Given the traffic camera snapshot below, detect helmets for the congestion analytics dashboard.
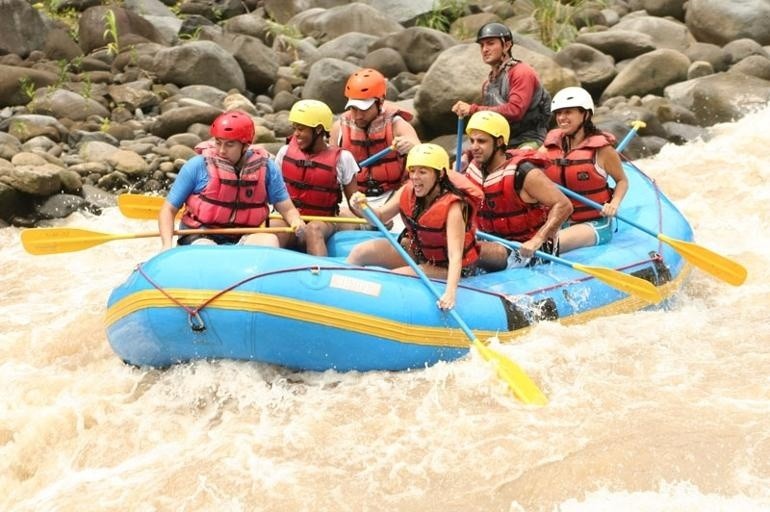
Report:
[465,110,510,167]
[208,111,255,168]
[288,99,334,154]
[549,86,596,139]
[344,67,387,129]
[406,143,450,199]
[475,21,514,65]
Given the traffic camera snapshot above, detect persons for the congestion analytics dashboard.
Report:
[329,68,423,197]
[342,140,483,312]
[508,84,632,252]
[459,109,577,273]
[449,20,556,153]
[155,108,306,252]
[261,98,395,260]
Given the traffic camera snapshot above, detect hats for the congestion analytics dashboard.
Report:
[344,96,378,111]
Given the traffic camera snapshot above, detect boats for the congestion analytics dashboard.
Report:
[105,154,699,375]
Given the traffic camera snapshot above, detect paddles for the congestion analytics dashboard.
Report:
[117,193,366,223]
[547,183,748,287]
[355,194,550,409]
[21,228,300,256]
[475,229,663,304]
